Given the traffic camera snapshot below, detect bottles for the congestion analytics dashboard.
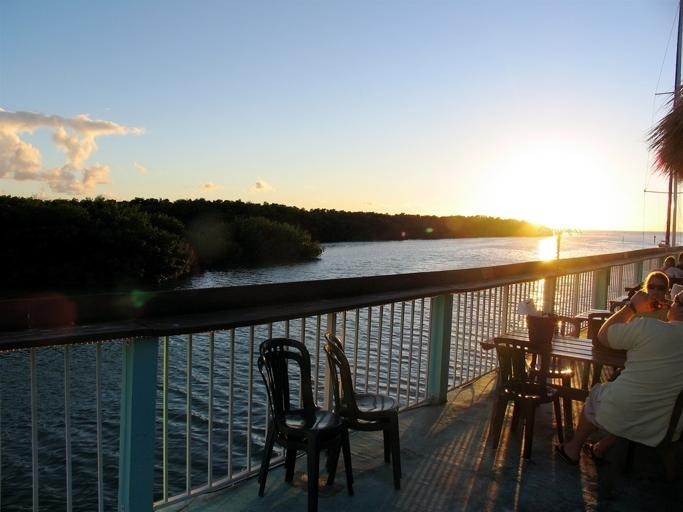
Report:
[628,288,670,314]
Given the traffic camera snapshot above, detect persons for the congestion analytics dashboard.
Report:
[661,256,682,279]
[676,253,682,269]
[623,270,672,324]
[550,285,682,468]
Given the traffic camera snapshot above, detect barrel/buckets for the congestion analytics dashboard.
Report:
[526,312,557,342]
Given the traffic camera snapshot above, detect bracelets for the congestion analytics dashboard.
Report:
[624,298,637,316]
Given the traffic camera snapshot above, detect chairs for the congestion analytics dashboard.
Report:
[256,333,403,511]
[490,275,682,480]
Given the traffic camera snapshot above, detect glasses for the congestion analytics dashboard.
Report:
[648,285,666,290]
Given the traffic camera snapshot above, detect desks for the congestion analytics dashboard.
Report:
[481,324,627,426]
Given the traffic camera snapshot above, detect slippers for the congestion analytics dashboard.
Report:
[583,441,602,464]
[556,442,577,463]
